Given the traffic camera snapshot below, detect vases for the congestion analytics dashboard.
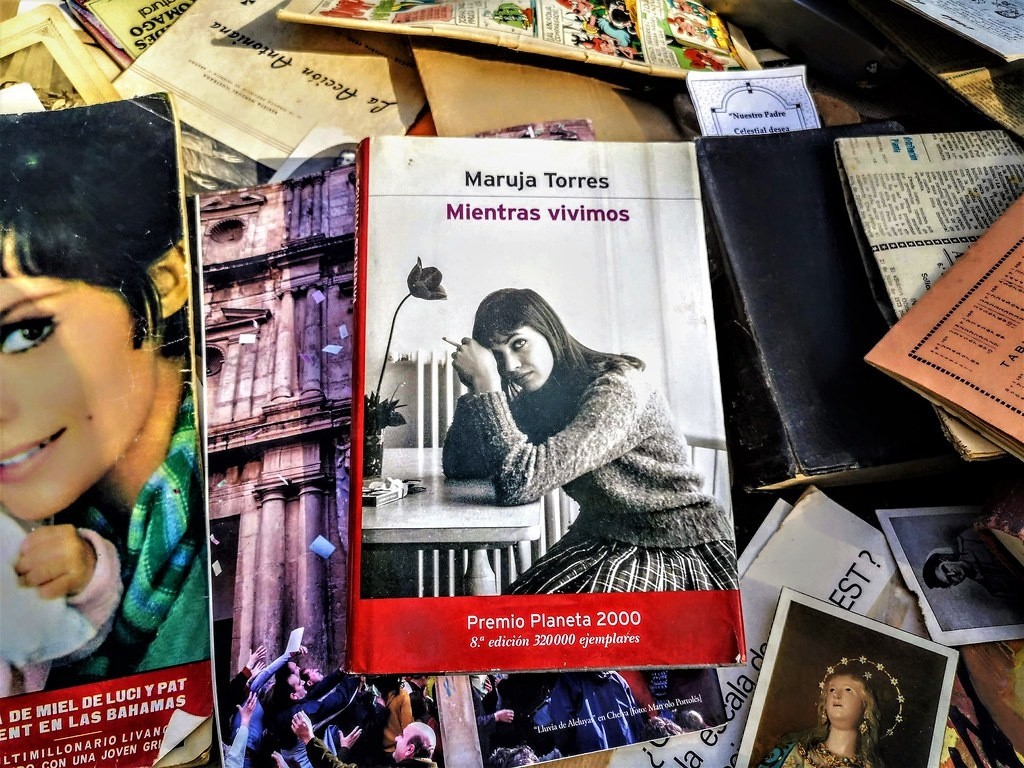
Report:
[362,431,384,487]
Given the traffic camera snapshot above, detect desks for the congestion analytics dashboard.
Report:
[363,448,543,597]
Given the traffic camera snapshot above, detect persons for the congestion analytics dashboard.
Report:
[221,645,442,768]
[0,115,209,698]
[471,673,708,768]
[335,149,357,167]
[444,292,739,595]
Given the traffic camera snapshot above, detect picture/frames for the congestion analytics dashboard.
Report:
[0,2,122,111]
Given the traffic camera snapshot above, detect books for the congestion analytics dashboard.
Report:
[346,135,747,674]
[691,128,1024,494]
[0,92,222,768]
[278,0,761,80]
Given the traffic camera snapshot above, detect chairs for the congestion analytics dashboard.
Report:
[686,434,734,529]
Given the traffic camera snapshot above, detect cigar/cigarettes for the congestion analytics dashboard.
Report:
[442,336,460,348]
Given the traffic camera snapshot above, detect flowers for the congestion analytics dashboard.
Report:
[363,255,448,437]
